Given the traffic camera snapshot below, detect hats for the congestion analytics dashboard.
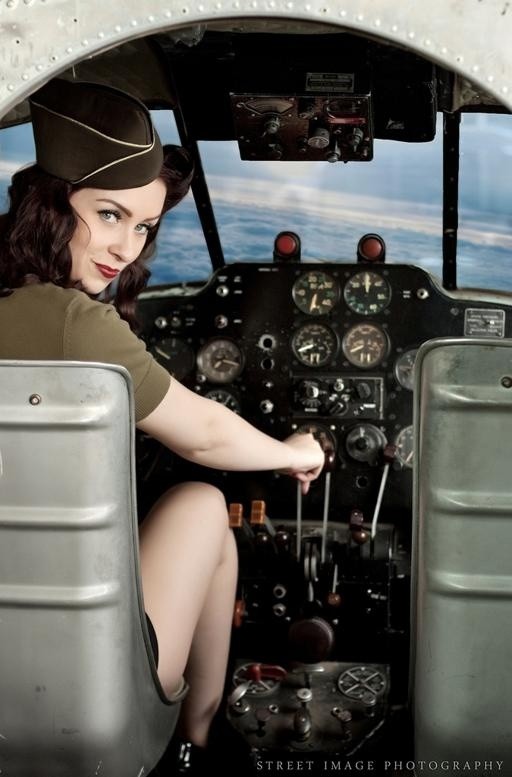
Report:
[27,75,165,194]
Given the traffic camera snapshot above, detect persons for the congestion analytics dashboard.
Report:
[1,76,325,776]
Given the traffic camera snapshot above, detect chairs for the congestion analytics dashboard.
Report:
[404,335,511,777]
[0,358,188,777]
